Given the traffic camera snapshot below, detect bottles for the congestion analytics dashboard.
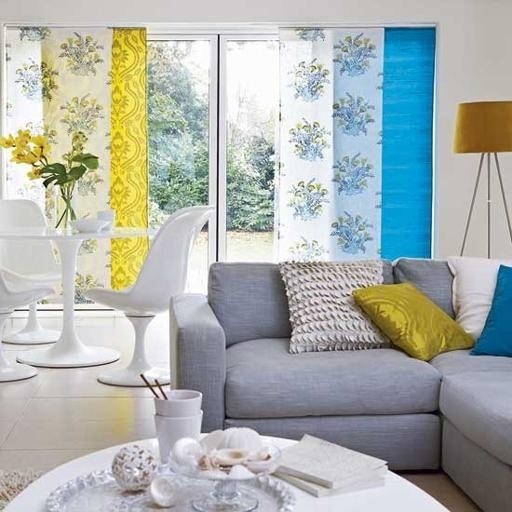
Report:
[112,443,157,494]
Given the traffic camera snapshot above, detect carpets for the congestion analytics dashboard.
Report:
[0,469,44,512]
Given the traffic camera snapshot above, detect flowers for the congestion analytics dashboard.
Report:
[1,125,103,228]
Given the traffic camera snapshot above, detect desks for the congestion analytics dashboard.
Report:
[0,227,159,370]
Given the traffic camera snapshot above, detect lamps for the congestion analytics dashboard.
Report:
[453,100,512,259]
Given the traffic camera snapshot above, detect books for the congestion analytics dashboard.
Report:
[269,432,389,497]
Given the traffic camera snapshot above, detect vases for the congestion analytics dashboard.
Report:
[52,180,79,234]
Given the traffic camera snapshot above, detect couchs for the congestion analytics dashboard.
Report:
[167,256,512,512]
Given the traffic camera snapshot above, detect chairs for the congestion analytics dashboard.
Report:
[0,198,63,346]
[82,204,217,388]
[0,265,57,384]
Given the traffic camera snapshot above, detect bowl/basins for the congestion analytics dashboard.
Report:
[74,218,107,232]
[148,478,181,506]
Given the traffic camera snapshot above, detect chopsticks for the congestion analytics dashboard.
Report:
[140,370,168,398]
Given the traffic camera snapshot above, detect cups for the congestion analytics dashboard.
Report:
[98,209,120,228]
[152,389,203,460]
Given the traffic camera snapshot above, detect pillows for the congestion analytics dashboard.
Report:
[276,259,397,354]
[352,282,475,361]
[446,257,512,344]
[469,264,512,358]
